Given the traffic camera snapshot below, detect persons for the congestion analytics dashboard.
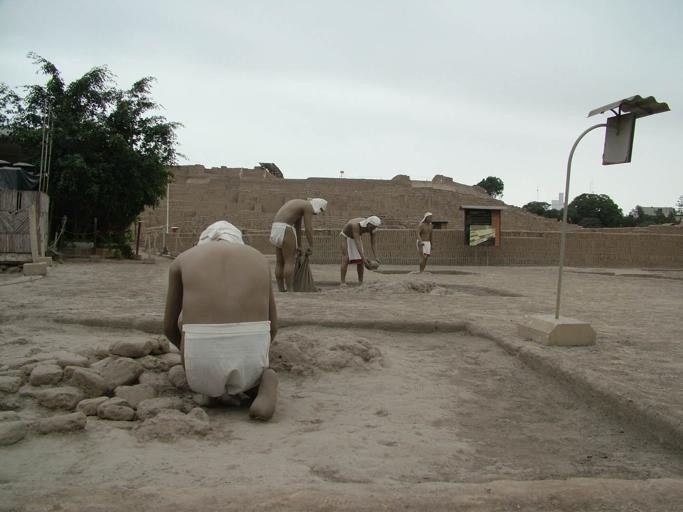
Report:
[337,216,381,288]
[268,197,327,292]
[162,219,278,422]
[415,212,433,272]
